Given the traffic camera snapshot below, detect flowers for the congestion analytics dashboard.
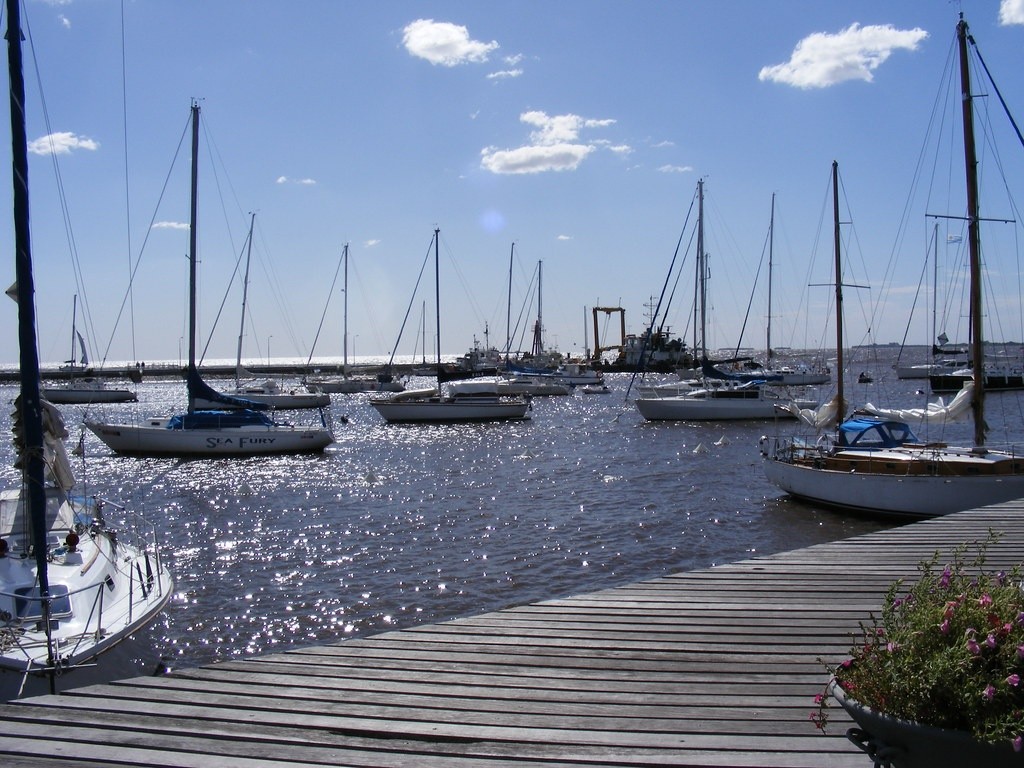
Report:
[809,525,1024,753]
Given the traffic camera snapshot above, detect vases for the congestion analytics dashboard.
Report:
[829,659,1024,768]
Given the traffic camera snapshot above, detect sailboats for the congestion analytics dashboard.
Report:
[368,177,834,425]
[893,213,1023,393]
[300,243,406,393]
[196,212,333,409]
[80,95,336,456]
[1,0,174,707]
[41,295,139,403]
[756,11,1023,519]
